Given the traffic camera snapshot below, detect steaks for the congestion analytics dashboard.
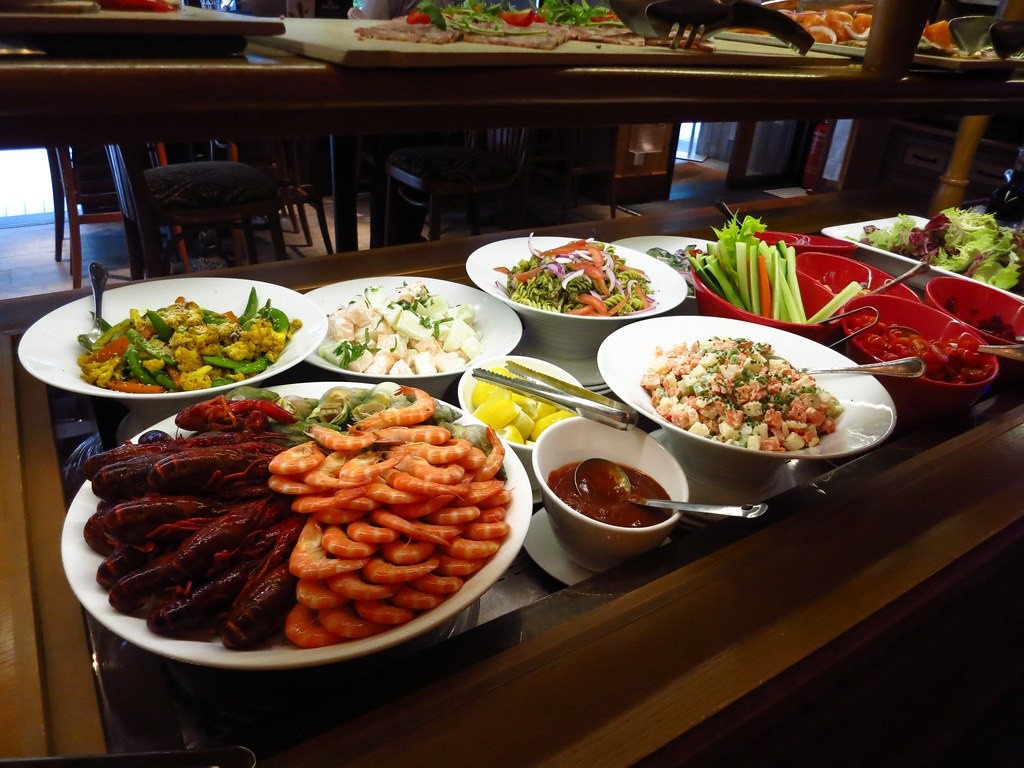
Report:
[349,10,716,51]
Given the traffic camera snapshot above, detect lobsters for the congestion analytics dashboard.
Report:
[82,395,299,652]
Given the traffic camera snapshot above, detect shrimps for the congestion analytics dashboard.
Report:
[270,385,508,647]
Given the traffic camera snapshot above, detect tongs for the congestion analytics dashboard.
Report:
[472,361,642,434]
[809,302,880,352]
[948,10,1024,61]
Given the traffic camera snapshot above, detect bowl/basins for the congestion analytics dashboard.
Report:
[455,356,590,493]
[594,313,900,519]
[531,412,691,575]
[691,211,1024,433]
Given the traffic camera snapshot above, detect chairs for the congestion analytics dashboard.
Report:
[49,144,123,288]
[101,140,294,271]
[379,126,537,240]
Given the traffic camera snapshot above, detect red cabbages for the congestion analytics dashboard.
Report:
[862,180,1024,302]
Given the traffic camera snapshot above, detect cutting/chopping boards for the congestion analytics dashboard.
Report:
[245,13,853,72]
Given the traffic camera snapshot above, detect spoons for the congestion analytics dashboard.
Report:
[887,323,1024,363]
[755,356,926,383]
[78,262,111,356]
[573,459,769,519]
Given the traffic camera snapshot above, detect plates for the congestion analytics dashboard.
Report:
[466,234,689,362]
[290,276,523,379]
[608,236,727,318]
[61,379,534,670]
[16,277,328,420]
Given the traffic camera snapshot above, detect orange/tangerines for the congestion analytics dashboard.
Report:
[727,10,954,48]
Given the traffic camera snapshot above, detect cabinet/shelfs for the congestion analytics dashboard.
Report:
[3,0,1024,768]
